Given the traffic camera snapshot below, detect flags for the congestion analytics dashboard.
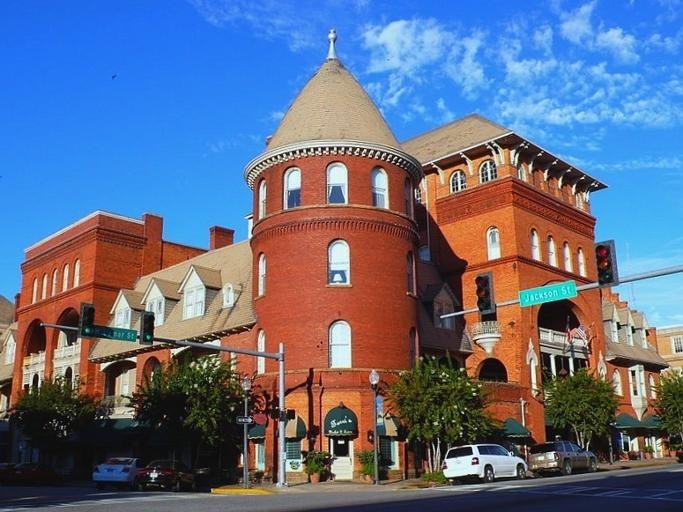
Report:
[566,320,586,344]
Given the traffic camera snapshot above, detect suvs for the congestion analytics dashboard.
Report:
[441,444,529,483]
[527,441,599,477]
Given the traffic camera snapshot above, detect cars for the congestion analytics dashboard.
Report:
[0,462,57,486]
[92,457,200,492]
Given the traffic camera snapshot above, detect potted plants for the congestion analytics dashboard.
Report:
[356,448,385,484]
[643,446,655,460]
[669,447,680,458]
[300,449,332,484]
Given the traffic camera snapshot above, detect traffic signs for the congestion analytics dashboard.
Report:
[102,326,137,342]
[518,280,577,308]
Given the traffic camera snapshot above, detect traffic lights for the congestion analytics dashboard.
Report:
[474,271,495,315]
[594,239,619,287]
[77,303,96,338]
[139,311,154,345]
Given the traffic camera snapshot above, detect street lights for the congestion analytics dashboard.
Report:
[368,369,380,485]
[241,378,252,488]
